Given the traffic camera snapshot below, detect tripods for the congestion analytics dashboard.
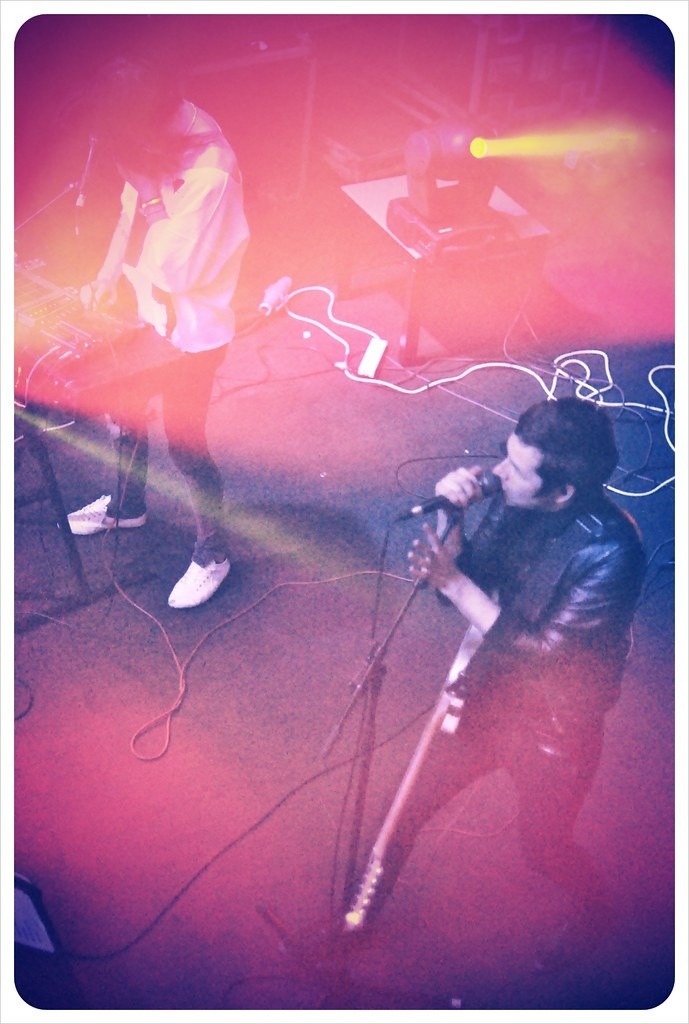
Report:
[250,504,467,1009]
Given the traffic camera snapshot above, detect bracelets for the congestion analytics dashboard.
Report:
[145,206,165,220]
[141,196,163,209]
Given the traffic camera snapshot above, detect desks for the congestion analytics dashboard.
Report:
[338,168,554,369]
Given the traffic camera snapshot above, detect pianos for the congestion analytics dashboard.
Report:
[14,261,186,423]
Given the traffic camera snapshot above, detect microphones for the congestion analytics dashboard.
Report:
[73,127,104,210]
[396,469,503,521]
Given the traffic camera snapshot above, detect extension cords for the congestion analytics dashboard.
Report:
[358,334,388,379]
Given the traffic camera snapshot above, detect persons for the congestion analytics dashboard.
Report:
[344,396,649,970]
[50,51,250,608]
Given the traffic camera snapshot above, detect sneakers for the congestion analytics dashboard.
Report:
[167,557,231,608]
[58,494,147,535]
